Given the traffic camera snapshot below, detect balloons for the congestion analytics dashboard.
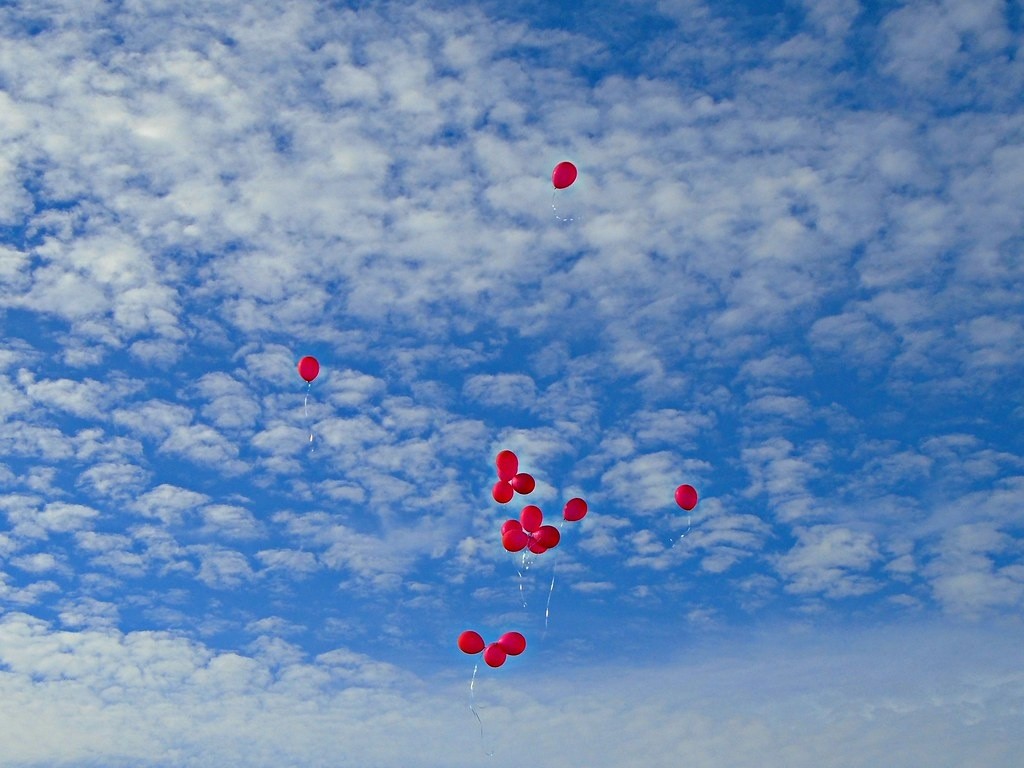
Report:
[492,482,514,503]
[526,533,548,555]
[553,161,578,190]
[496,450,519,479]
[484,643,507,667]
[497,469,518,482]
[520,505,543,533]
[498,631,526,656]
[533,525,560,549]
[502,529,528,552]
[564,498,588,521]
[510,473,535,494]
[675,484,698,511]
[501,520,522,535]
[298,356,320,383]
[458,630,485,654]
